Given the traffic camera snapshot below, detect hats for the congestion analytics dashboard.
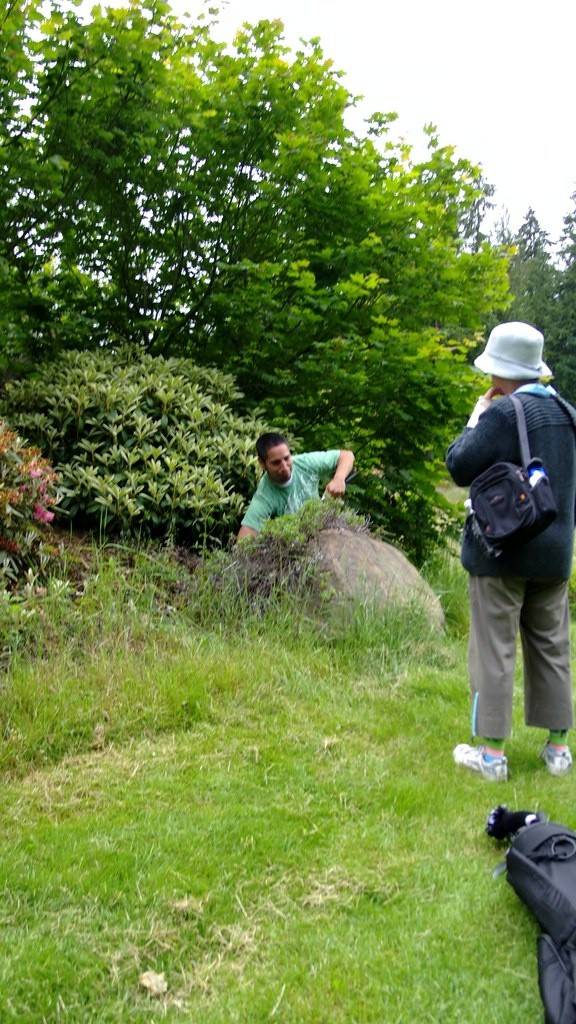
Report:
[474,321,552,380]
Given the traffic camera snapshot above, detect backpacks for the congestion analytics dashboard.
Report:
[506,817,576,1024]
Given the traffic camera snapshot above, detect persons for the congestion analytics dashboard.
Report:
[445,322,576,783]
[235,432,356,544]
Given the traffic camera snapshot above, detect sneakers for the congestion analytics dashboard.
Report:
[539,739,572,777]
[453,744,507,782]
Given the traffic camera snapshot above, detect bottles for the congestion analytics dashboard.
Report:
[529,466,556,514]
[463,498,496,560]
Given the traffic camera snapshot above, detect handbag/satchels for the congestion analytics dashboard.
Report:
[463,391,556,561]
